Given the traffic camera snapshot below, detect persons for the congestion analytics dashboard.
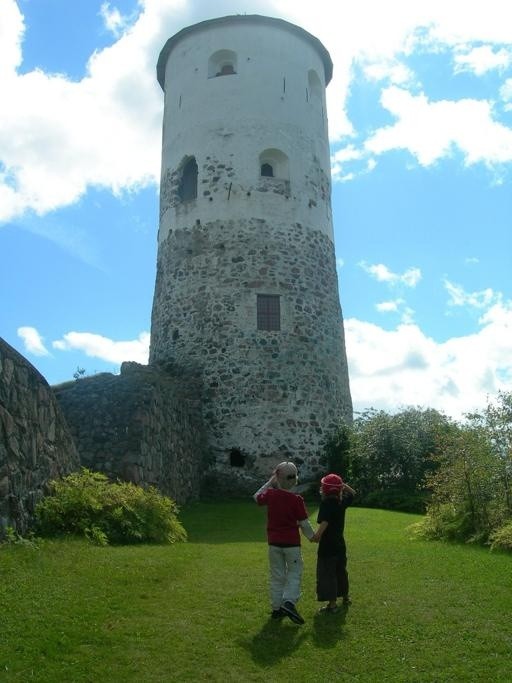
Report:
[315,474,356,613]
[253,462,315,624]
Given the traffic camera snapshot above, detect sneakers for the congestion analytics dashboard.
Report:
[279,601,306,625]
[270,609,287,619]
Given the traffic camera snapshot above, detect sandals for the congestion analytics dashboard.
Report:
[317,605,340,616]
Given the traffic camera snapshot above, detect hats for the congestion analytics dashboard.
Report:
[320,473,344,494]
[274,459,298,490]
